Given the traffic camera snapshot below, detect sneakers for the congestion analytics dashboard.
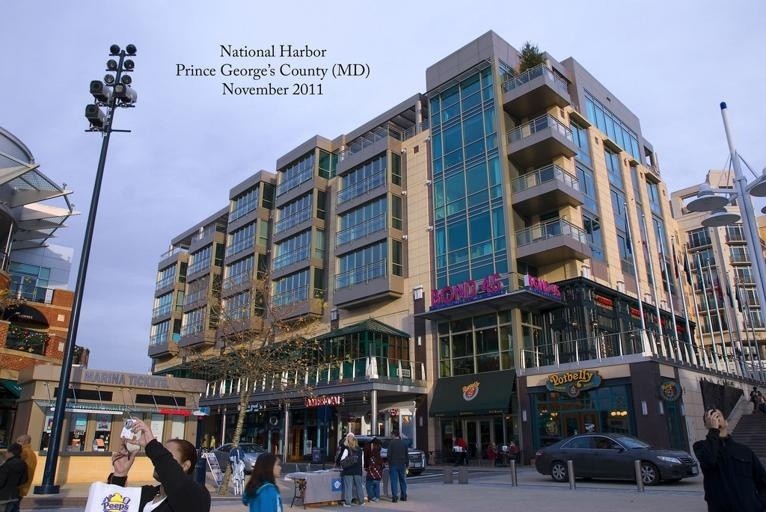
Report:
[343,496,409,508]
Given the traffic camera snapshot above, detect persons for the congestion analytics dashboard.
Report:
[339,437,365,506]
[241,452,283,512]
[0,442,29,512]
[331,433,353,470]
[454,434,469,465]
[208,434,215,452]
[508,439,520,455]
[199,433,207,449]
[691,408,766,512]
[106,416,210,511]
[749,386,766,414]
[487,444,496,469]
[365,438,382,503]
[13,434,37,504]
[386,430,409,503]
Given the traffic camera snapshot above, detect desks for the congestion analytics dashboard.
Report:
[288,470,367,509]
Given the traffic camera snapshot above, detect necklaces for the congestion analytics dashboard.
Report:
[154,491,159,497]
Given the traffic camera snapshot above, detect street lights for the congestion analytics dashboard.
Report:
[34,44,137,495]
[686,101,766,329]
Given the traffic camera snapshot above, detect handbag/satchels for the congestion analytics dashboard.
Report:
[339,454,360,470]
[84,472,143,512]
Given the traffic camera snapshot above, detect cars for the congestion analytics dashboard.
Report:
[205,443,266,473]
[337,435,425,476]
[535,432,699,485]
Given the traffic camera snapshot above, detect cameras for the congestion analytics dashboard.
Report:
[120,419,142,442]
